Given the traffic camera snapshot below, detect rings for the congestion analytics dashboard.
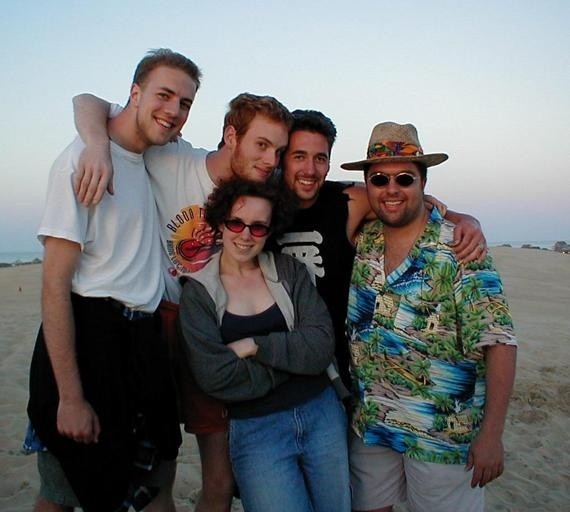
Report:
[478,241,484,253]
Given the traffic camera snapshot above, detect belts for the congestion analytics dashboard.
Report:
[109,300,152,323]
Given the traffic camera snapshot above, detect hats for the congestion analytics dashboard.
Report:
[340,122,449,170]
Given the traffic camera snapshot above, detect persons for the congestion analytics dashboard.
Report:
[70,89,448,510]
[17,46,206,510]
[177,176,354,511]
[260,108,488,407]
[337,120,519,510]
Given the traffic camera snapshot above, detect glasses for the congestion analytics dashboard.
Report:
[222,218,274,238]
[366,171,421,188]
